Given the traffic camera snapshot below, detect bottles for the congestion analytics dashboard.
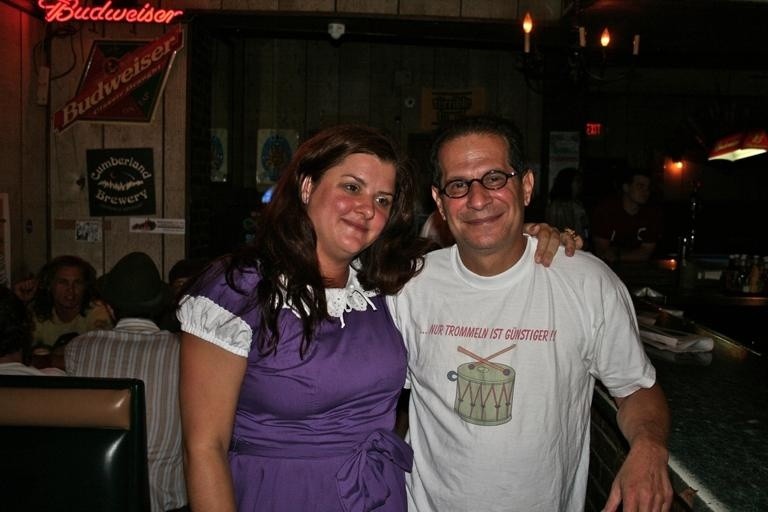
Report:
[726,253,768,298]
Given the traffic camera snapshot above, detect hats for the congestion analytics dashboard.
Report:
[94,253,172,316]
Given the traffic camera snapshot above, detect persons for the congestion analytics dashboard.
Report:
[2,251,215,512]
[416,207,458,252]
[542,164,593,250]
[375,109,683,511]
[167,121,584,511]
[586,164,671,264]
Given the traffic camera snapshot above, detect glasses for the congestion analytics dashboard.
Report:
[436,171,517,199]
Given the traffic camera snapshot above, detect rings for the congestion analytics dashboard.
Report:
[569,229,578,240]
